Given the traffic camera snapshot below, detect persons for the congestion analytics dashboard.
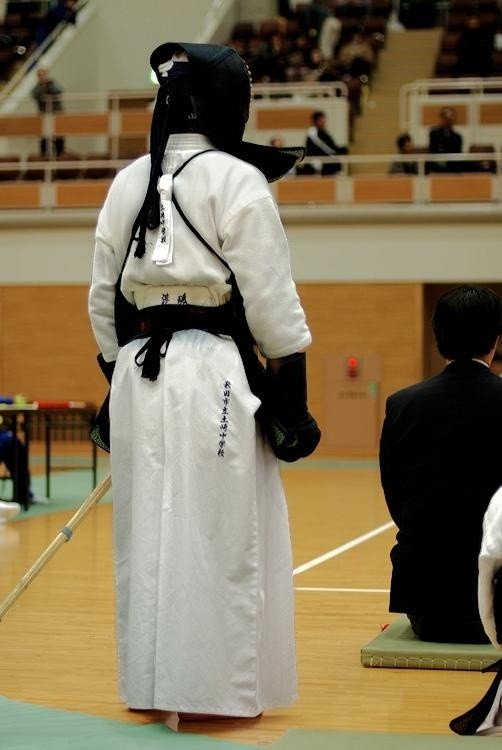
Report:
[375,278,502,648]
[302,106,350,178]
[269,134,301,179]
[0,0,86,83]
[425,106,463,173]
[87,43,323,725]
[224,0,502,142]
[0,397,52,512]
[390,133,415,175]
[28,67,70,158]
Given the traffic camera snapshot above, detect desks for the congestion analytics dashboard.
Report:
[0,406,98,497]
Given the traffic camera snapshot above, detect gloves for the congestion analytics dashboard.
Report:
[255,352,321,463]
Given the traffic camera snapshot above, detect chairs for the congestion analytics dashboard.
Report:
[0,3,502,228]
